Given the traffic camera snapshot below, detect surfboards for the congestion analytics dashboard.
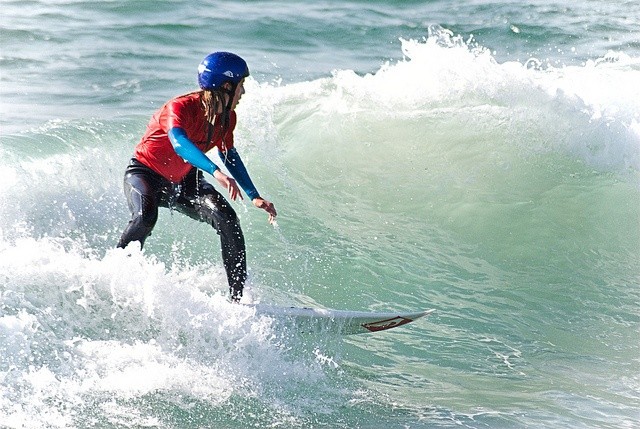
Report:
[242,304,437,335]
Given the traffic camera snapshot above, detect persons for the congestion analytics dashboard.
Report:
[117,52,276,303]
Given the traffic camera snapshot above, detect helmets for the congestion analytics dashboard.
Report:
[197,52,249,89]
